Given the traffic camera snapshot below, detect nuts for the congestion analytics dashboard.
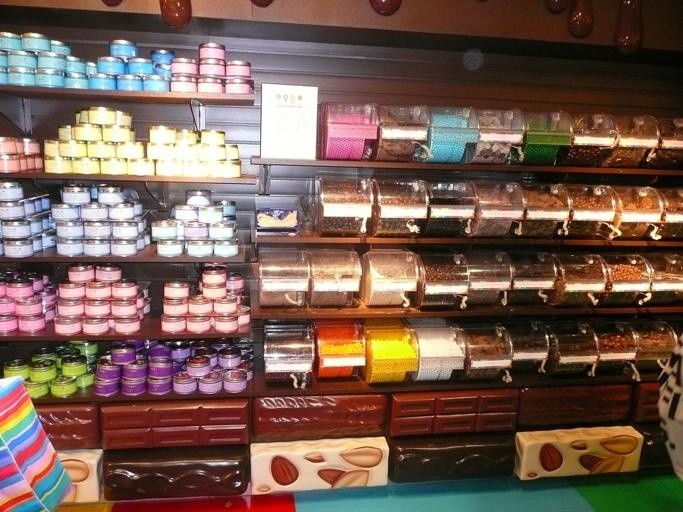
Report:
[60,459,89,482]
[339,446,383,467]
[257,485,270,492]
[539,442,563,472]
[527,472,537,477]
[600,435,638,454]
[63,484,77,502]
[571,442,587,450]
[271,456,299,486]
[318,469,369,489]
[304,452,325,463]
[579,454,624,475]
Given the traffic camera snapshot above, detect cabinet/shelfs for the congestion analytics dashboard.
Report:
[1,80,253,505]
[252,73,683,498]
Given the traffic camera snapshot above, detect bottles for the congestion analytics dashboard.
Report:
[51,183,150,258]
[94,339,191,396]
[3,340,98,399]
[170,43,255,95]
[151,190,239,258]
[172,337,254,394]
[160,266,251,334]
[146,125,241,179]
[0,181,57,259]
[44,106,155,176]
[0,31,176,93]
[0,266,59,333]
[53,262,152,336]
[0,136,44,174]
